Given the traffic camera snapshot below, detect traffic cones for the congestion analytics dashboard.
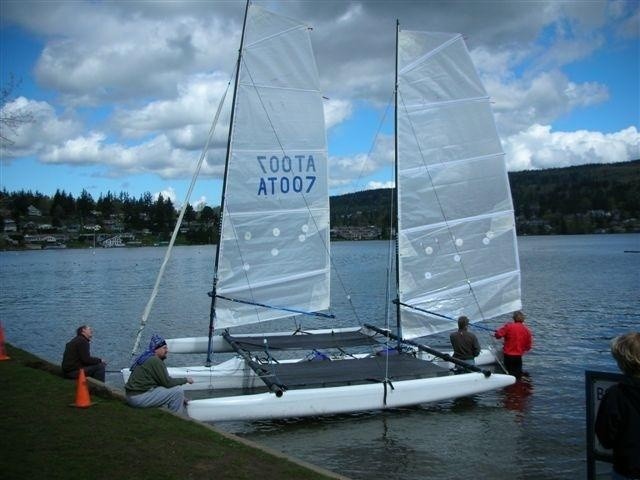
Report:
[0,319,11,361]
[68,369,98,408]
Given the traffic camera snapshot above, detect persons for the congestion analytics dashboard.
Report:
[61,325,108,383]
[123,334,195,418]
[594,332,640,480]
[449,316,481,366]
[491,311,532,372]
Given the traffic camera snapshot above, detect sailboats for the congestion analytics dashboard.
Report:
[121,0,525,425]
